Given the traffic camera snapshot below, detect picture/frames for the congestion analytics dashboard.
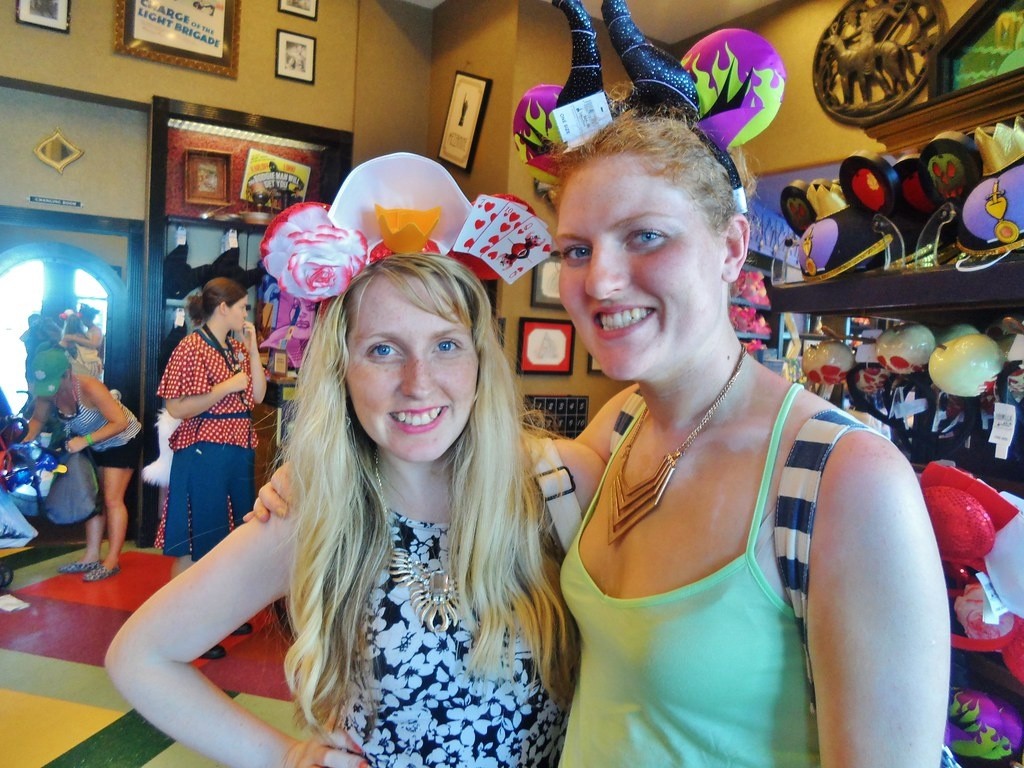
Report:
[275,28,316,84]
[438,69,493,173]
[532,250,567,311]
[14,0,71,34]
[184,147,235,206]
[114,0,242,79]
[242,148,312,212]
[277,0,319,21]
[517,315,576,375]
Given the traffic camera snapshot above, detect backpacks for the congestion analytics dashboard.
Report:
[72,337,103,376]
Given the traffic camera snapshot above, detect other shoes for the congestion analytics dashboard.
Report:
[200,645,226,659]
[231,623,252,635]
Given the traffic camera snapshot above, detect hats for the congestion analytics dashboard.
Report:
[31,348,70,396]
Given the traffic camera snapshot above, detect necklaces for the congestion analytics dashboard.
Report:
[603,344,747,541]
[373,452,462,635]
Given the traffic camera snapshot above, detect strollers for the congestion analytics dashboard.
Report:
[0,418,38,587]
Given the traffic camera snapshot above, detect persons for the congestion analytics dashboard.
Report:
[20,304,109,430]
[512,0,954,768]
[19,347,144,582]
[156,278,292,634]
[103,157,604,768]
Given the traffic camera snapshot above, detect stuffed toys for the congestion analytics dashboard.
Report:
[781,116,1024,280]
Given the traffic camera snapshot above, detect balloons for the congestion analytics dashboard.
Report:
[800,323,1004,396]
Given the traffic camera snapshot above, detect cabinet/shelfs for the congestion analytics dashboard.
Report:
[728,264,775,340]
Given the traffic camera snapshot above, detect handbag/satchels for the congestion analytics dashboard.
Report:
[42,434,104,528]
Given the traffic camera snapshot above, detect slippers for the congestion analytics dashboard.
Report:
[83,564,120,582]
[57,560,99,573]
